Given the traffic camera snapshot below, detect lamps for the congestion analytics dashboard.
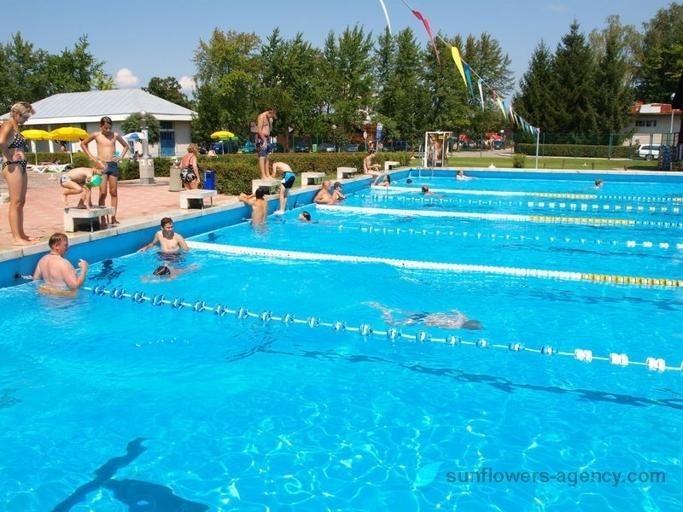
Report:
[25,160,71,175]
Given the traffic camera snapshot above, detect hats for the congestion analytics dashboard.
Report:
[319,142,333,153]
[242,141,256,155]
[381,140,409,153]
[296,142,310,153]
[268,142,283,153]
[343,143,357,153]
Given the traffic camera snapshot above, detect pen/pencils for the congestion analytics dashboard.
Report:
[494,141,505,150]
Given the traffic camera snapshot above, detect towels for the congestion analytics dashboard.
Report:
[168,166,182,192]
[661,146,676,171]
[203,170,214,190]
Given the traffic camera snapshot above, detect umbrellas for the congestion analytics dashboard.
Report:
[210,131,234,155]
[123,132,146,153]
[49,127,90,163]
[20,129,49,165]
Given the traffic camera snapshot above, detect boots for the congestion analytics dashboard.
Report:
[212,140,239,154]
[636,143,676,163]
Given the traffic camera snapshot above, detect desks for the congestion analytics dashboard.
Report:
[499,129,505,149]
[331,124,336,153]
[670,93,676,134]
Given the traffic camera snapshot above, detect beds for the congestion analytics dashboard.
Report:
[49,127,90,163]
[210,131,234,155]
[20,129,49,165]
[123,132,146,153]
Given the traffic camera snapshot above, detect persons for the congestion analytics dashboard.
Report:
[60,144,474,284]
[593,180,602,189]
[0,101,37,246]
[255,108,277,181]
[81,117,128,227]
[371,302,483,329]
[32,233,88,289]
